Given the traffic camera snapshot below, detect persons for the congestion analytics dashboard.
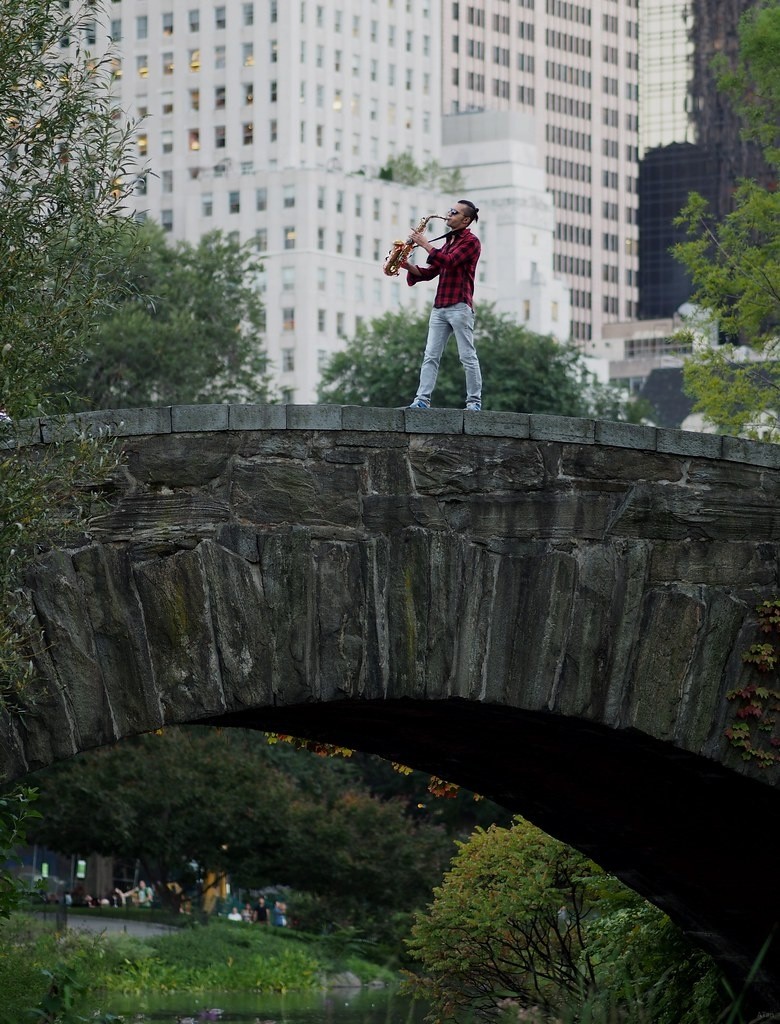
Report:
[47,880,191,914]
[556,906,569,927]
[396,200,482,411]
[229,898,287,927]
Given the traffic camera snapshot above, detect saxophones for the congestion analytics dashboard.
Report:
[382,214,450,277]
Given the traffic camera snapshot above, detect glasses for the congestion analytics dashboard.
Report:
[449,208,467,218]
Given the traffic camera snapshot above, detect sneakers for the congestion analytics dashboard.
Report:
[464,403,481,410]
[410,395,431,408]
